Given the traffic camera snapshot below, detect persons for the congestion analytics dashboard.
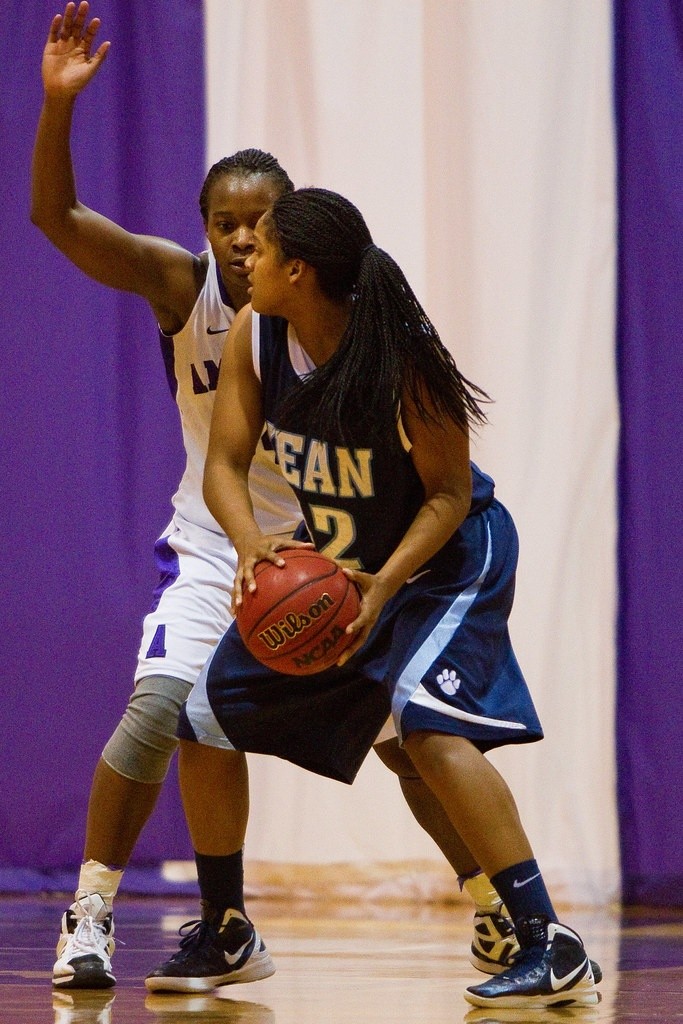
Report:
[143,189,605,1010]
[27,0,602,989]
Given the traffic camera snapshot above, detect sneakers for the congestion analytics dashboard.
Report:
[145,899,276,994]
[469,902,603,984]
[463,913,598,1010]
[53,893,117,990]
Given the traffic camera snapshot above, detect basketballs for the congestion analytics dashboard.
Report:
[233,549,362,678]
